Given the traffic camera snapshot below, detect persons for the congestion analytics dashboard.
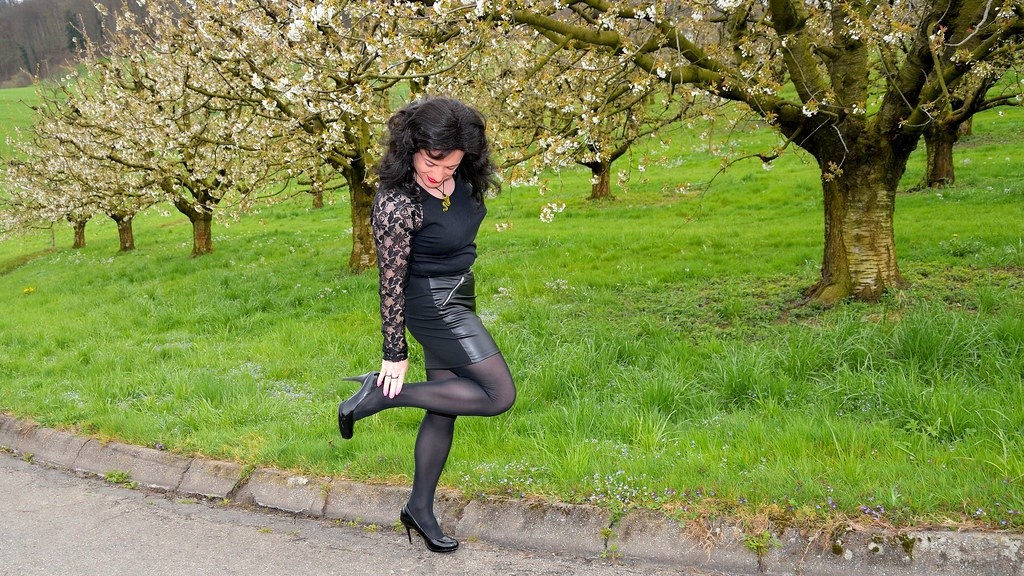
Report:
[337,97,517,553]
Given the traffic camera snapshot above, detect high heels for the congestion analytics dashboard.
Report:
[338,371,385,439]
[400,503,459,553]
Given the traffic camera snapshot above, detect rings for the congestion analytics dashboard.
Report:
[385,375,391,376]
[391,377,398,379]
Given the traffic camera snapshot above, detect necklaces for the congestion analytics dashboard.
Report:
[434,182,450,212]
